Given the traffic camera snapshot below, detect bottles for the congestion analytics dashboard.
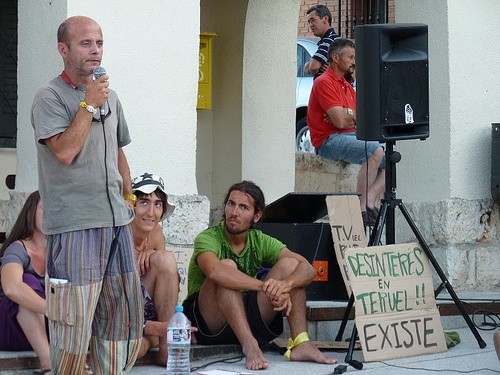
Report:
[166,306,192,375]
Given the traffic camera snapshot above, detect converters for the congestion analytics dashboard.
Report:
[334,364,347,373]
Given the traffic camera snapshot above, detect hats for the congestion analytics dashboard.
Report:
[130,172,175,222]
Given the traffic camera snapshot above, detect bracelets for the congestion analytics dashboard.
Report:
[123,192,138,207]
[79,101,97,115]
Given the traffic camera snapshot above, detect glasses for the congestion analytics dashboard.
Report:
[89,97,111,124]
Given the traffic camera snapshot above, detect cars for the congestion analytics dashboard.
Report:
[295,38,359,156]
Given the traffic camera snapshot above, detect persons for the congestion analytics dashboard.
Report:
[0,190,93,375]
[305,38,399,227]
[130,172,199,366]
[302,3,354,85]
[29,15,145,375]
[181,180,338,370]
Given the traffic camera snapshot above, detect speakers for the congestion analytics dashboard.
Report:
[353,22,430,141]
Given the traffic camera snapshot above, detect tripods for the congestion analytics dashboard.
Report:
[336,143,486,364]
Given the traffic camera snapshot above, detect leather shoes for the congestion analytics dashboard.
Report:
[362,206,386,226]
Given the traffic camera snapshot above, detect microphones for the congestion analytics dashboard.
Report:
[94,67,106,122]
